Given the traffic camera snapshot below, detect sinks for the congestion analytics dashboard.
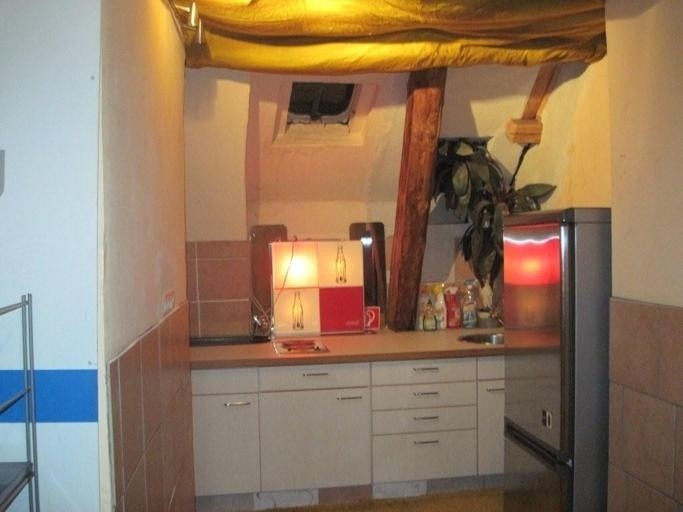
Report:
[458,331,506,346]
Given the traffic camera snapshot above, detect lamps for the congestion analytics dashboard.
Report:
[361,231,372,246]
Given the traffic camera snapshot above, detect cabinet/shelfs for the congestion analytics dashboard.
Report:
[258,361,372,492]
[370,356,478,484]
[191,366,260,496]
[478,355,505,476]
[0,294,40,512]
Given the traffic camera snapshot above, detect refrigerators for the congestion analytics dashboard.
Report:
[500,206,611,511]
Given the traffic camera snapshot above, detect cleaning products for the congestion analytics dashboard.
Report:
[463,285,477,327]
[425,298,436,330]
[444,288,461,328]
[427,282,448,330]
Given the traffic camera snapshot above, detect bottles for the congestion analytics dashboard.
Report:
[335,245,347,284]
[422,299,437,331]
[292,291,304,330]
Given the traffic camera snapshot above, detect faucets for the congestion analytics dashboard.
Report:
[491,311,503,328]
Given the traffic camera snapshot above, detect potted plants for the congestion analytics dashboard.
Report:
[433,143,557,328]
